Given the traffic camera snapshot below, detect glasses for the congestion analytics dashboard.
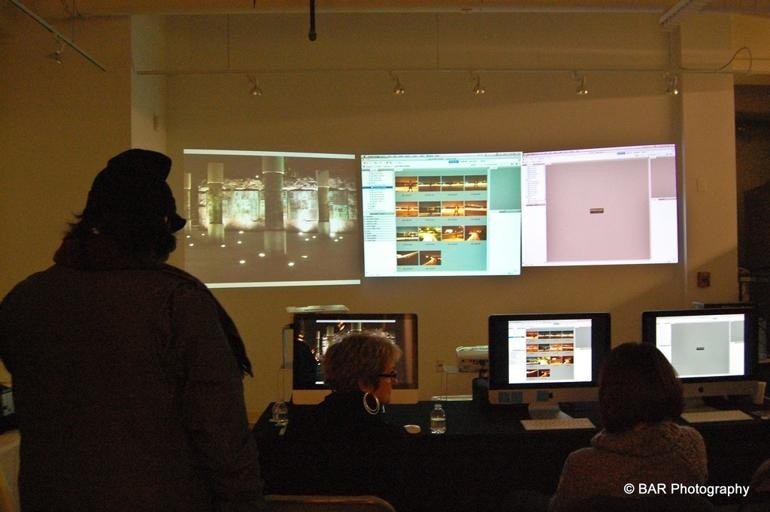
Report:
[380,370,398,382]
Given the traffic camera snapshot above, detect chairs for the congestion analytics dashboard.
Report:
[575,495,714,512]
[264,494,396,511]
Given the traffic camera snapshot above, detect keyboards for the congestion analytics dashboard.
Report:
[680,410,753,424]
[519,417,596,432]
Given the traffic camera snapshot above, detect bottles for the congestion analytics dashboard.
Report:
[430,403,448,434]
[272,399,289,422]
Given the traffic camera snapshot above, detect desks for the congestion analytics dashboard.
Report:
[252,402,769,512]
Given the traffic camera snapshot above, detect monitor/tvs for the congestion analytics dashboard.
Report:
[488,313,611,418]
[642,308,759,411]
[292,312,419,405]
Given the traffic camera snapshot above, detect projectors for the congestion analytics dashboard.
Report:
[456,344,488,367]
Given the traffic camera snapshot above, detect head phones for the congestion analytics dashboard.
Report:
[157,190,172,229]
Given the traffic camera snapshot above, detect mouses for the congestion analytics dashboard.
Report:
[403,424,421,434]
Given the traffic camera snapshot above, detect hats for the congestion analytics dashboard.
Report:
[81,148,186,234]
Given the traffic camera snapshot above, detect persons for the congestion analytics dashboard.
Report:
[294,319,319,387]
[550,341,713,511]
[0,150,267,511]
[281,331,444,511]
